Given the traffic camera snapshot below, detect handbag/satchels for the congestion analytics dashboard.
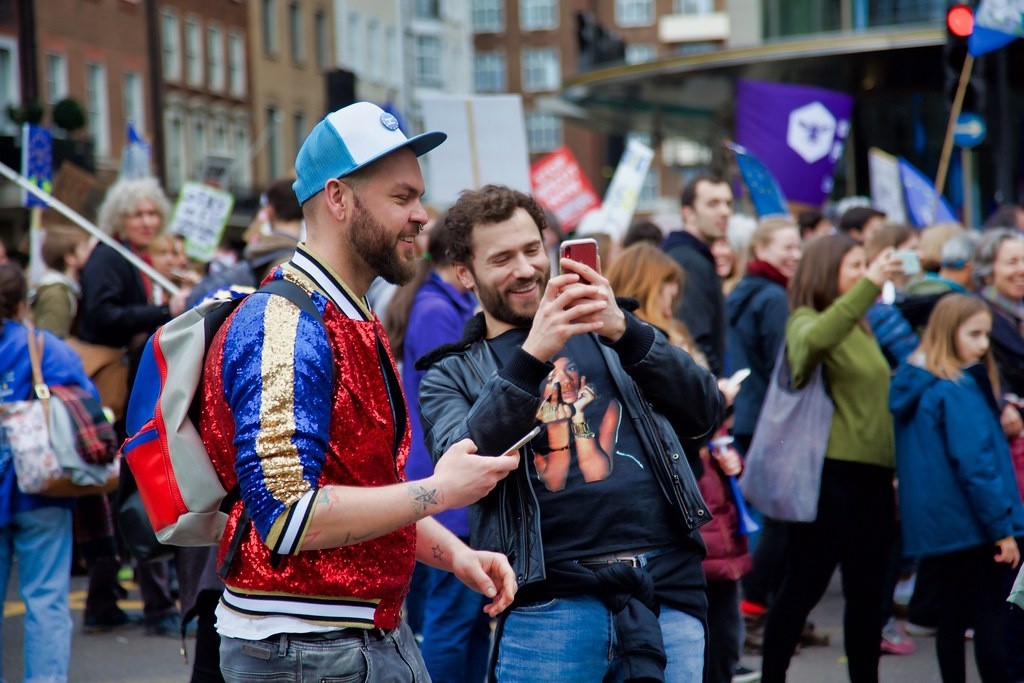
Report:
[708,444,759,534]
[736,336,832,522]
[0,326,121,498]
[63,335,133,423]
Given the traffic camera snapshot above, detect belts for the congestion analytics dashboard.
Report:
[580,550,673,573]
[269,627,396,644]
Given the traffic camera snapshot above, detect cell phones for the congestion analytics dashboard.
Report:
[501,427,541,457]
[729,368,751,385]
[890,251,922,275]
[560,238,597,285]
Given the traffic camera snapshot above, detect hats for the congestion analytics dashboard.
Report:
[291,101,447,207]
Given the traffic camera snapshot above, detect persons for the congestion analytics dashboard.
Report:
[368,207,495,683]
[0,176,303,683]
[201,101,520,683]
[418,184,726,683]
[582,174,1024,683]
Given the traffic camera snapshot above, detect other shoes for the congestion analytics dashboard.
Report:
[82,611,146,635]
[800,624,829,646]
[145,610,198,638]
[904,621,936,637]
[879,634,915,654]
[71,563,94,576]
[742,626,800,656]
[117,563,134,580]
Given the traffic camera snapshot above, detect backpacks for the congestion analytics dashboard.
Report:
[124,278,323,548]
